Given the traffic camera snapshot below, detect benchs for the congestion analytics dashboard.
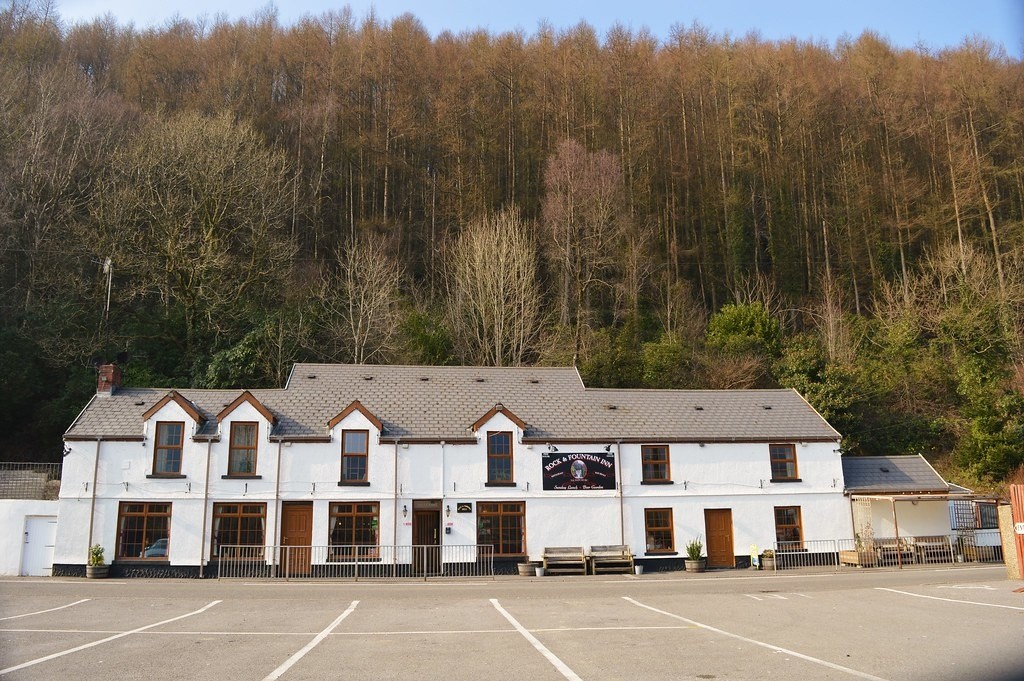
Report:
[589,545,636,575]
[541,546,587,576]
[873,535,952,565]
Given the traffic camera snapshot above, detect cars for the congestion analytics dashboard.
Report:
[139,539,169,557]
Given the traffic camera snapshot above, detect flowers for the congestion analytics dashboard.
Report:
[89,543,106,566]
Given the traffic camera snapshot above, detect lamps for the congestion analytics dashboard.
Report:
[545,442,558,451]
[833,448,847,455]
[604,440,617,452]
[446,505,451,517]
[403,504,408,517]
[63,445,71,457]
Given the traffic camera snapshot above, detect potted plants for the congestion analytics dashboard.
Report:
[685,533,706,572]
[517,556,540,576]
[762,549,775,569]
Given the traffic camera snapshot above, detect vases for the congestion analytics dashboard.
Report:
[635,564,643,575]
[86,565,109,578]
[535,567,545,576]
